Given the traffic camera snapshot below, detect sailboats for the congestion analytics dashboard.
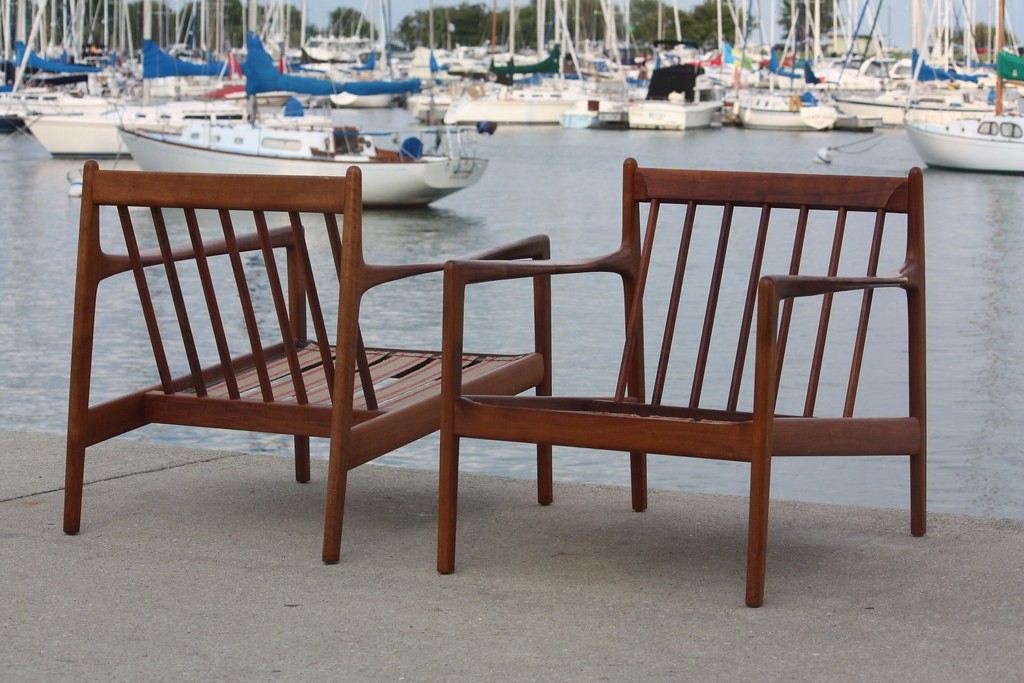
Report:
[0,2,1022,172]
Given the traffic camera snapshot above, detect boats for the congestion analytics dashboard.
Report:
[113,112,492,207]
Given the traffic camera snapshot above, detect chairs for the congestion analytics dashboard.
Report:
[63,160,555,566]
[436,157,927,609]
